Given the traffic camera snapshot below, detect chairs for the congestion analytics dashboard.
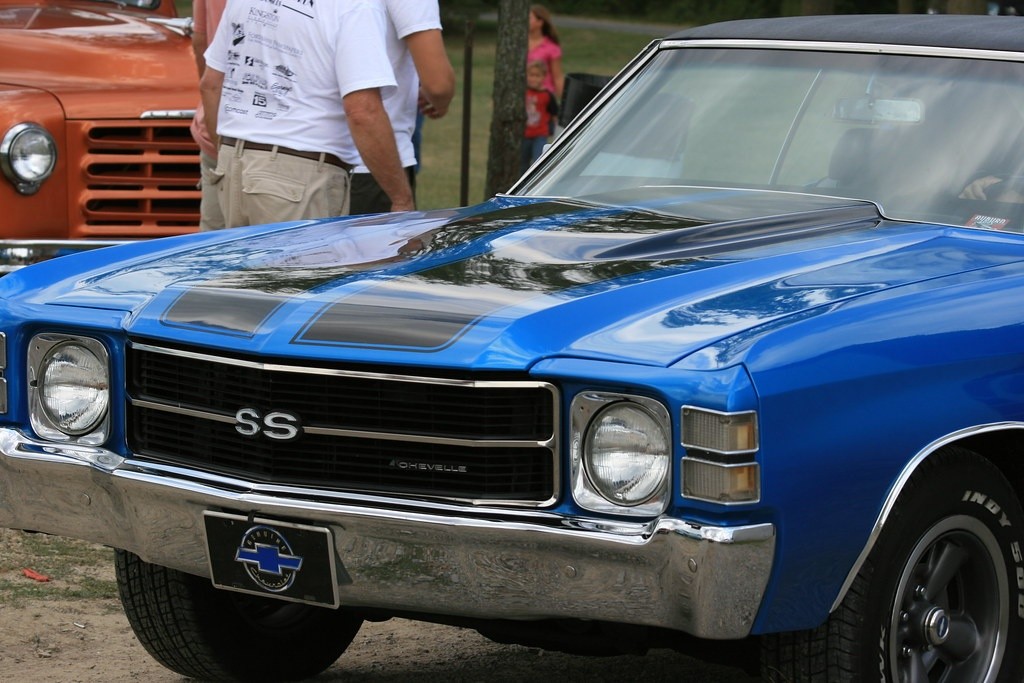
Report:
[818,128,904,195]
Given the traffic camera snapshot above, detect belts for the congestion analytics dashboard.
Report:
[216,136,357,174]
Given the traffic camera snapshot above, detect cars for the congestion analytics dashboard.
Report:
[0,12,1024,683]
[0,1,206,273]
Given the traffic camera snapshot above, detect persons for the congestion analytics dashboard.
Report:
[958,144,1024,205]
[189,0,566,233]
